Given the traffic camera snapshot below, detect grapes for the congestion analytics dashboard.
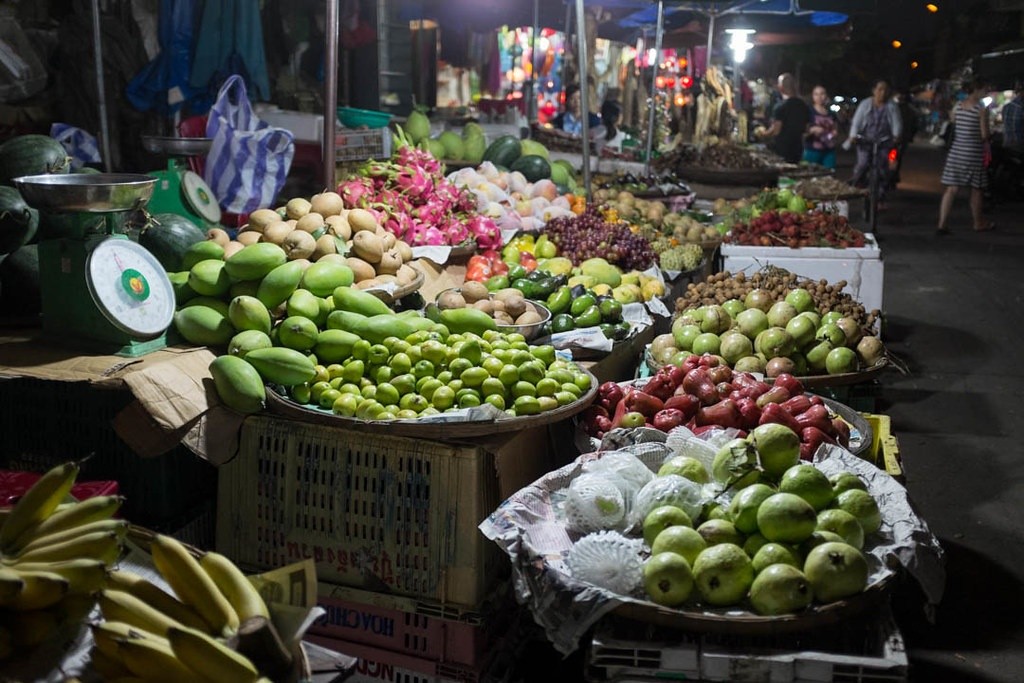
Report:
[540,201,657,273]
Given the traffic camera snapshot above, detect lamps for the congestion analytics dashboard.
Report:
[724,13,757,63]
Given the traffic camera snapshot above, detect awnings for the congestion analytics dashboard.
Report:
[566,0,857,149]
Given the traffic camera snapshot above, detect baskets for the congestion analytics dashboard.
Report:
[320,126,392,160]
[215,413,521,683]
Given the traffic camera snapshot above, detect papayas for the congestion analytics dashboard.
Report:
[405,95,489,163]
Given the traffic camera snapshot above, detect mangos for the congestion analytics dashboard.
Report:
[167,240,498,413]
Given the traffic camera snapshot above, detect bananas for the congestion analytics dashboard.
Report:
[1,461,293,683]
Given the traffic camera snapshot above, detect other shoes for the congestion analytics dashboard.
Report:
[936,226,949,234]
[973,219,998,232]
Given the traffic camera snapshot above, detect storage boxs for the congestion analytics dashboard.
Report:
[720,229,884,338]
[321,126,390,163]
[204,375,908,682]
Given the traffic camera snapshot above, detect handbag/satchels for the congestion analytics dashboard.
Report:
[202,74,295,216]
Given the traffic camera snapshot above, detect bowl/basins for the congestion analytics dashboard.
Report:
[436,288,550,343]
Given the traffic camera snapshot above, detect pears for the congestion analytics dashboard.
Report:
[566,422,884,615]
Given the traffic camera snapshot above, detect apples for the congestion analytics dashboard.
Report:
[463,249,536,283]
[651,286,885,376]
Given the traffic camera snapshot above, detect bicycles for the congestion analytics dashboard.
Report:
[853,133,894,235]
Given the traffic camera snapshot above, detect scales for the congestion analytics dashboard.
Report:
[14,172,176,359]
[136,133,226,234]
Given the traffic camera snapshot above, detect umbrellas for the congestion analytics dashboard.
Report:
[912,58,996,97]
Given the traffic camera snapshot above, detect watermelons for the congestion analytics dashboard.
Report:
[0,133,208,306]
[481,135,572,195]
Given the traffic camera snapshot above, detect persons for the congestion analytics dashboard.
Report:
[934,78,997,236]
[800,83,842,176]
[1001,88,1024,163]
[588,99,645,155]
[756,72,812,164]
[840,78,904,212]
[546,83,603,139]
[892,88,917,188]
